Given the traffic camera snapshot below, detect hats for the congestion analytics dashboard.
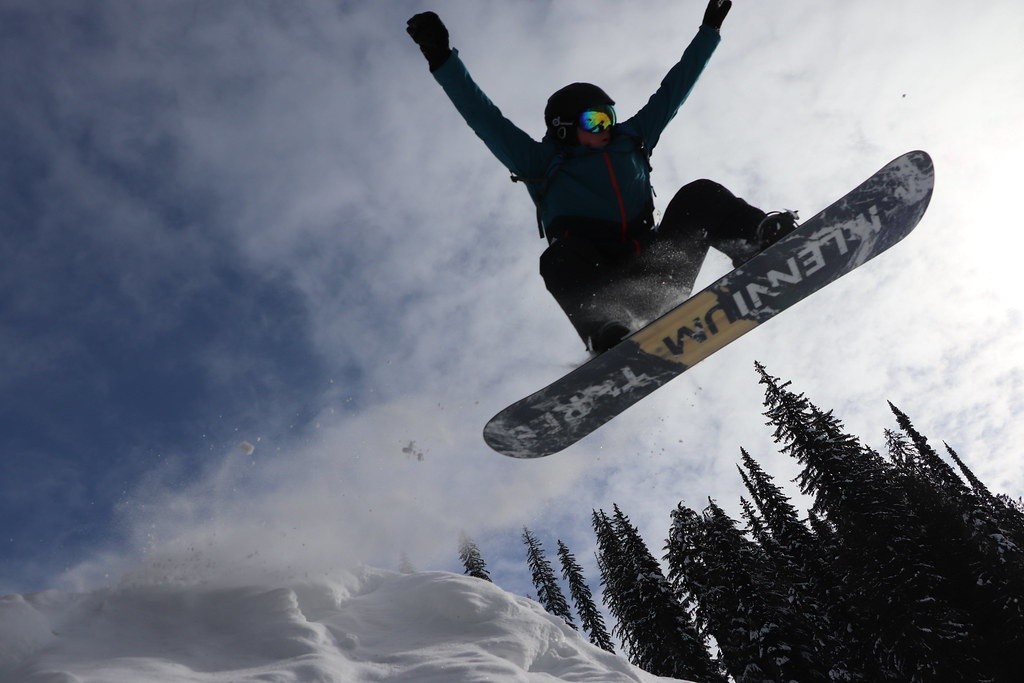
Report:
[543,82,616,146]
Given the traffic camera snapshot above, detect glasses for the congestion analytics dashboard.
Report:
[578,101,617,135]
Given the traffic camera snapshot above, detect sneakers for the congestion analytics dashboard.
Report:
[732,211,795,267]
[587,320,632,355]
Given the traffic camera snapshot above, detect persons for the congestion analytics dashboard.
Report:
[405,0,798,356]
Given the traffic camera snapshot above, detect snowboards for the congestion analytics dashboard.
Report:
[482,149,937,461]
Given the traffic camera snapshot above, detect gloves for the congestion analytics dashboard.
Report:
[406,12,454,73]
[698,1,733,32]
